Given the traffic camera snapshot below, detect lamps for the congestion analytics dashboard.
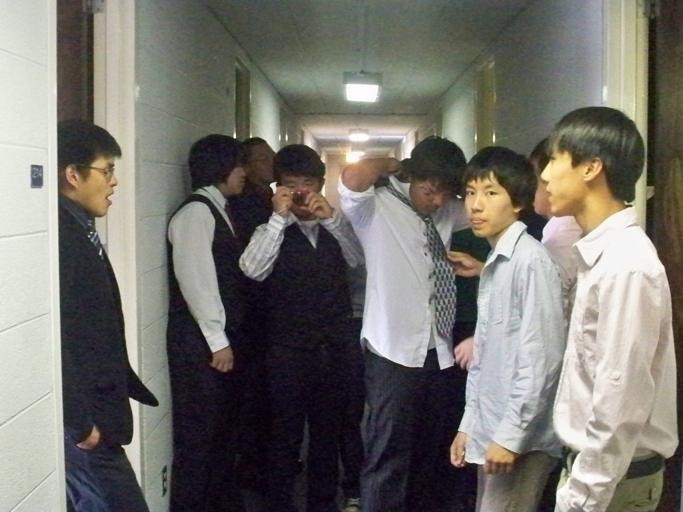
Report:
[341,71,382,102]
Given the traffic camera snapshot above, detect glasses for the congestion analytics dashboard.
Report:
[64,163,117,183]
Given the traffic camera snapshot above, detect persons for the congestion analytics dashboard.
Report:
[538,105,679,511]
[236,136,274,246]
[162,133,250,511]
[336,134,470,512]
[57,119,163,512]
[236,142,368,512]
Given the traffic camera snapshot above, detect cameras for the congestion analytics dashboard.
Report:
[288,191,310,207]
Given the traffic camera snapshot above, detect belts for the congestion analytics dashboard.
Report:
[559,446,665,481]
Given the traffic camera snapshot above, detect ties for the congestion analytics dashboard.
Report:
[225,203,239,239]
[384,183,458,339]
[304,224,317,247]
[85,218,105,263]
